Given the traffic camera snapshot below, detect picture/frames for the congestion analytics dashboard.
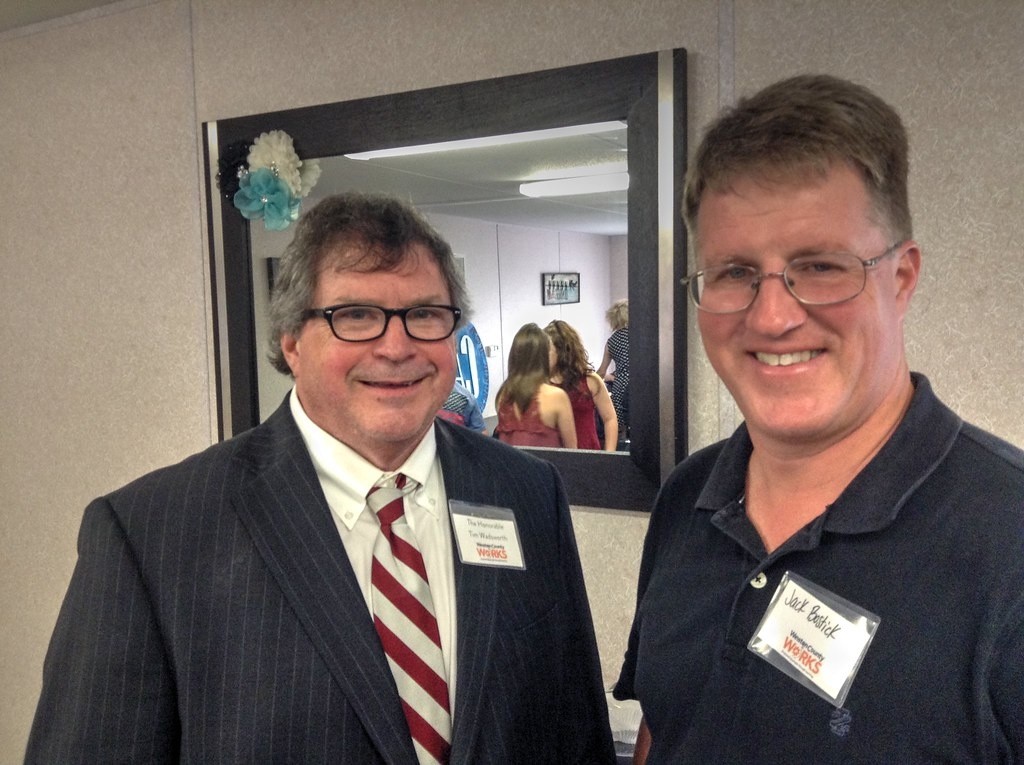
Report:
[542,271,580,305]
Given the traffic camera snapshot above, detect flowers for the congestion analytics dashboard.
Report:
[229,128,303,229]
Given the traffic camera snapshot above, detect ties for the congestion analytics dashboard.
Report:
[366,472,453,764]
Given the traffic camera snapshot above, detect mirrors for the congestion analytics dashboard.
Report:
[200,48,687,515]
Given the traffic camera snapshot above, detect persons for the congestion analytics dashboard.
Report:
[612,72,1024,765]
[436,297,630,453]
[21,189,619,765]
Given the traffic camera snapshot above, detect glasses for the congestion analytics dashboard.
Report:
[302,303,462,341]
[681,241,898,313]
